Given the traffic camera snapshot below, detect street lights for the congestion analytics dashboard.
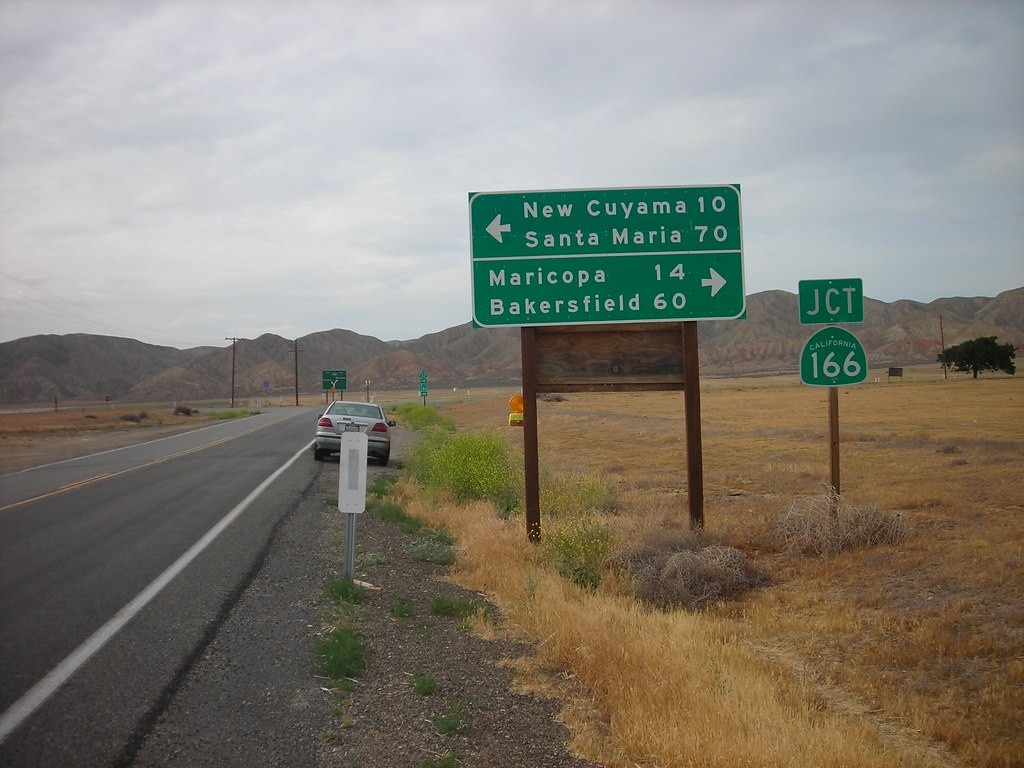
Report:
[288,348,305,406]
[225,337,236,407]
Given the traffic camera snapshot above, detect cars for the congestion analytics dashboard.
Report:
[314,400,397,468]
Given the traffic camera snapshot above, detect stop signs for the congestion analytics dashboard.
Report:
[104,396,110,402]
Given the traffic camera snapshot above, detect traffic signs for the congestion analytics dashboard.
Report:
[467,183,747,328]
